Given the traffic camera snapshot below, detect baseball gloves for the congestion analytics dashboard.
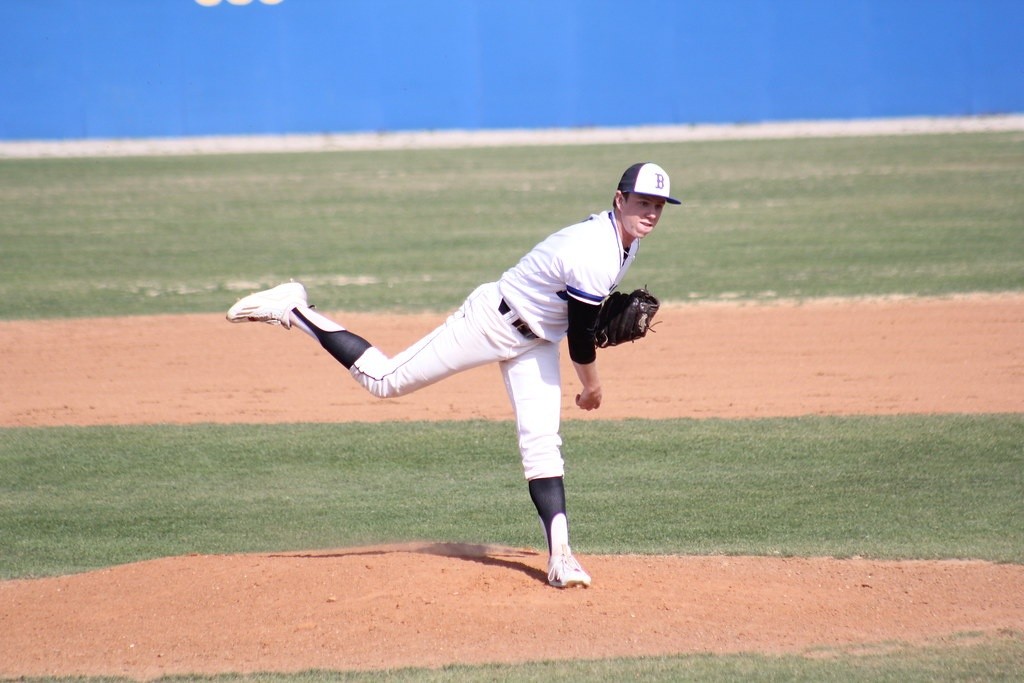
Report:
[594,288,661,349]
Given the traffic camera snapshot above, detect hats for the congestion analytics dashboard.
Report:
[616,162,681,204]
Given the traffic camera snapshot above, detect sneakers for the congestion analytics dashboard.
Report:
[226,282,316,330]
[548,544,591,589]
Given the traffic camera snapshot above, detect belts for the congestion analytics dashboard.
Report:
[499,298,540,338]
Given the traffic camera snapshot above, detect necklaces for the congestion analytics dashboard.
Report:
[623,249,636,261]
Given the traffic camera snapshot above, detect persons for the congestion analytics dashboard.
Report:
[225,162,681,589]
